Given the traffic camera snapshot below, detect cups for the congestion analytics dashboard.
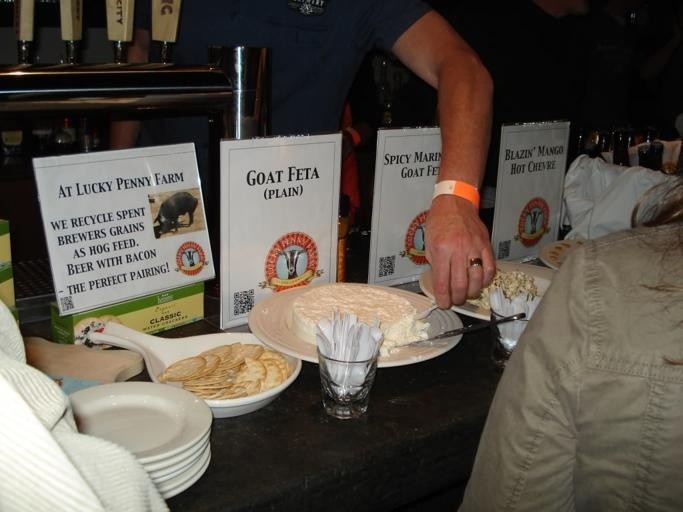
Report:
[317,346,377,424]
[489,307,530,371]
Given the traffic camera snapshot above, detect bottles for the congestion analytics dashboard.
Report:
[51,114,103,156]
[574,120,664,172]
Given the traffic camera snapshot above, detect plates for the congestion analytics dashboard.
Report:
[248,283,464,369]
[539,239,587,270]
[68,382,213,501]
[419,260,556,322]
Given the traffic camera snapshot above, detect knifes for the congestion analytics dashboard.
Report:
[391,313,526,348]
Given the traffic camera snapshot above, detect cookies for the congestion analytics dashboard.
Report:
[159,343,291,401]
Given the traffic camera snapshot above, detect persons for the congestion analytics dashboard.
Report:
[457,1,683,511]
[108,1,496,308]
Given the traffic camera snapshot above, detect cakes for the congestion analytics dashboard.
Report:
[287,283,427,351]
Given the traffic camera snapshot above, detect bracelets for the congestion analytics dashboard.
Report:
[433,181,481,209]
